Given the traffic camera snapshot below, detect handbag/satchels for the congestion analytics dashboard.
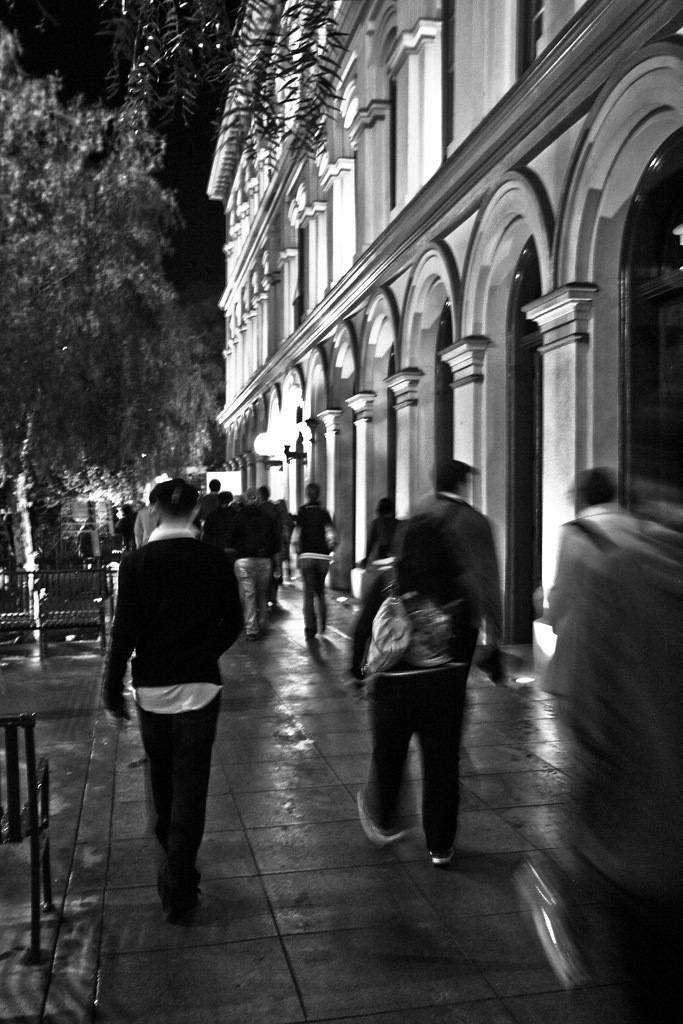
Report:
[367,592,412,674]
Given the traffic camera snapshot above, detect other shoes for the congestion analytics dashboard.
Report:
[261,629,268,638]
[246,634,261,641]
[357,795,402,842]
[429,846,455,864]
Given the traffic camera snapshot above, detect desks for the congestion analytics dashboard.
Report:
[0,654,105,966]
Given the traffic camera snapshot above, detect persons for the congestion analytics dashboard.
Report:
[396,460,502,780]
[115,479,339,642]
[544,422,683,1024]
[104,479,244,923]
[348,517,481,865]
[356,498,396,570]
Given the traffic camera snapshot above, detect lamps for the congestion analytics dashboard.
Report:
[254,431,283,468]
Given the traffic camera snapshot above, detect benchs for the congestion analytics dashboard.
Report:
[0,566,120,656]
